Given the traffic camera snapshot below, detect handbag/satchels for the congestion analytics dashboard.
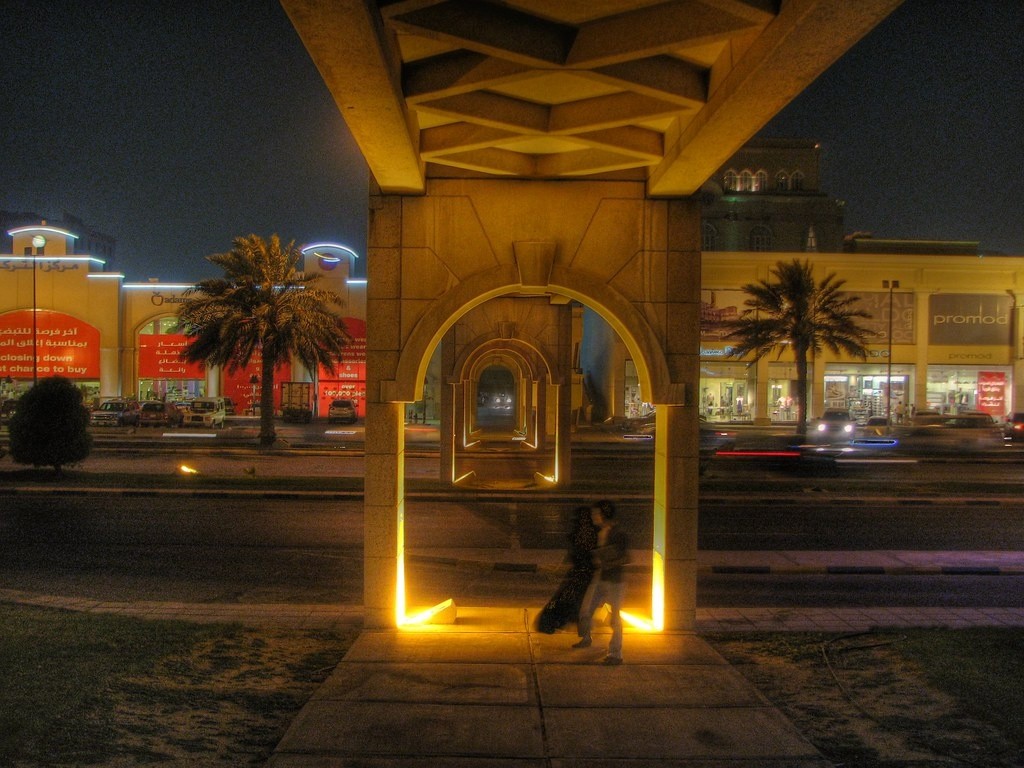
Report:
[591,544,619,561]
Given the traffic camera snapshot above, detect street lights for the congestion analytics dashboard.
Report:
[880,280,901,437]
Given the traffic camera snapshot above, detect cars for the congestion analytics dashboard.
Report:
[90,394,239,430]
[1002,410,1024,441]
[816,407,857,438]
[863,415,891,439]
[905,409,941,436]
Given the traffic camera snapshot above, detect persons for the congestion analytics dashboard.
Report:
[904,404,908,414]
[572,499,631,662]
[895,401,904,423]
[911,403,915,417]
[707,392,714,415]
[776,396,793,412]
[735,395,744,417]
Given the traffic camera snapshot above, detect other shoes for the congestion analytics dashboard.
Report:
[572,638,592,647]
[595,655,623,666]
